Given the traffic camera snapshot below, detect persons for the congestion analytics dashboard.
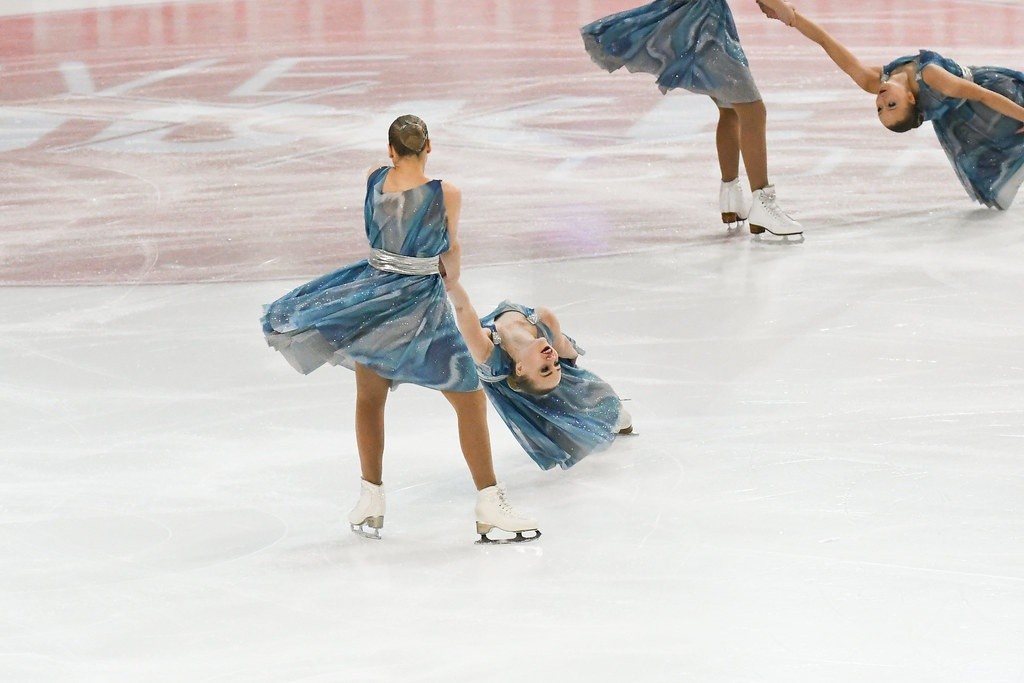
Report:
[441,271,635,471]
[257,116,544,542]
[796,4,1024,209]
[582,0,805,249]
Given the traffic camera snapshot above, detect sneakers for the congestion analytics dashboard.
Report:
[619,405,639,435]
[749,185,803,235]
[718,176,753,232]
[348,476,386,540]
[474,482,541,545]
